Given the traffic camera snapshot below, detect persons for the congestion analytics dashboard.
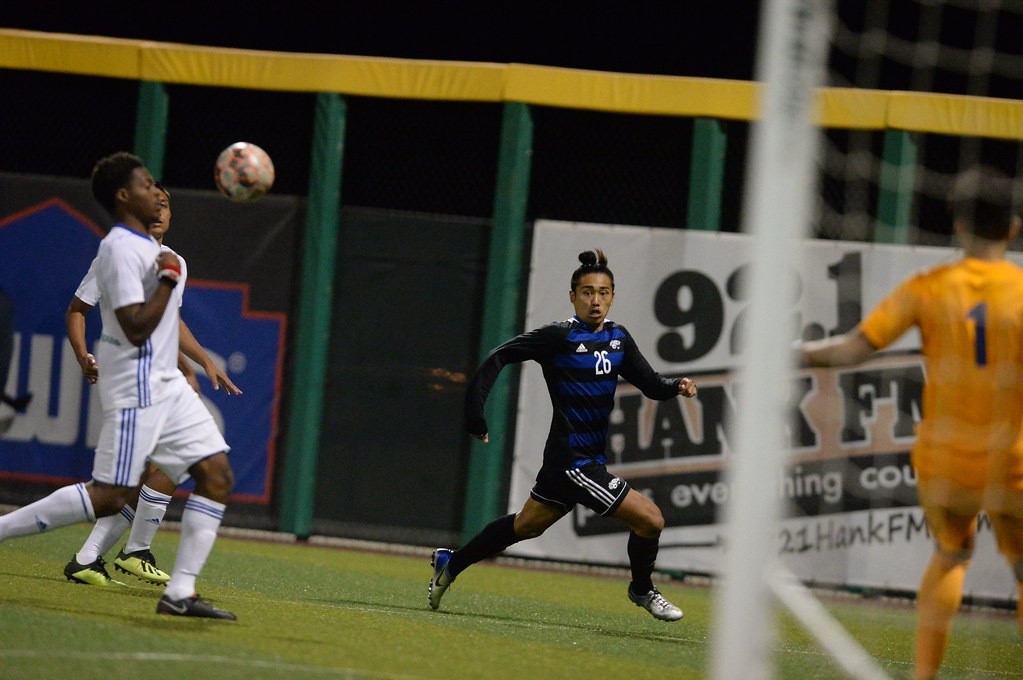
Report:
[427,249,698,621]
[0,152,243,622]
[794,167,1023,680]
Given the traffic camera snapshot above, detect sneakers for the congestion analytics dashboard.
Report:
[64,553,126,587]
[428,549,454,609]
[156,593,236,619]
[115,544,170,586]
[627,580,684,622]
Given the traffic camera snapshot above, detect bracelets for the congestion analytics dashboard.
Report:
[157,266,180,286]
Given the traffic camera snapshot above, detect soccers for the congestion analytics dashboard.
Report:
[214,141,276,205]
